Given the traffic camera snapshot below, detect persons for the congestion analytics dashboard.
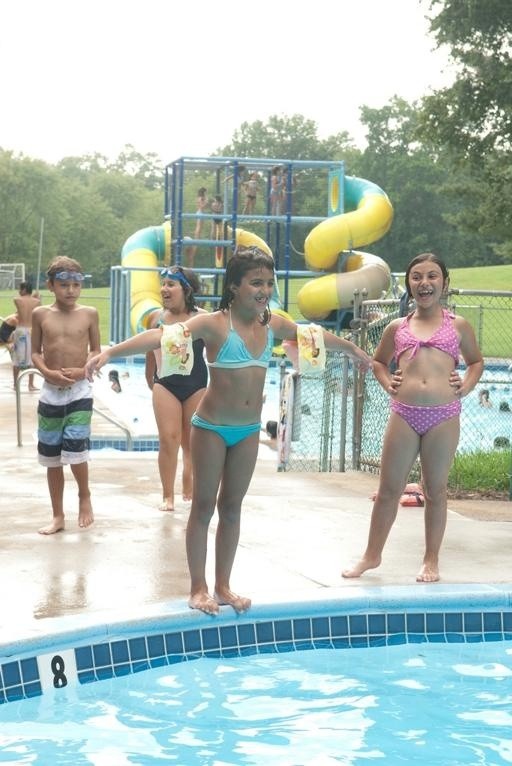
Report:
[83,235,371,615]
[194,187,209,240]
[170,236,198,267]
[479,388,511,448]
[108,369,123,393]
[221,161,297,215]
[341,251,483,583]
[145,265,210,512]
[30,256,102,535]
[0,281,42,391]
[211,196,223,240]
[259,378,309,454]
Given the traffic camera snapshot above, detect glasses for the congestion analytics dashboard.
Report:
[55,272,86,283]
[160,268,190,287]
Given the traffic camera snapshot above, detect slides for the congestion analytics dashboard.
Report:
[119,221,171,335]
[225,225,294,355]
[295,170,392,343]
